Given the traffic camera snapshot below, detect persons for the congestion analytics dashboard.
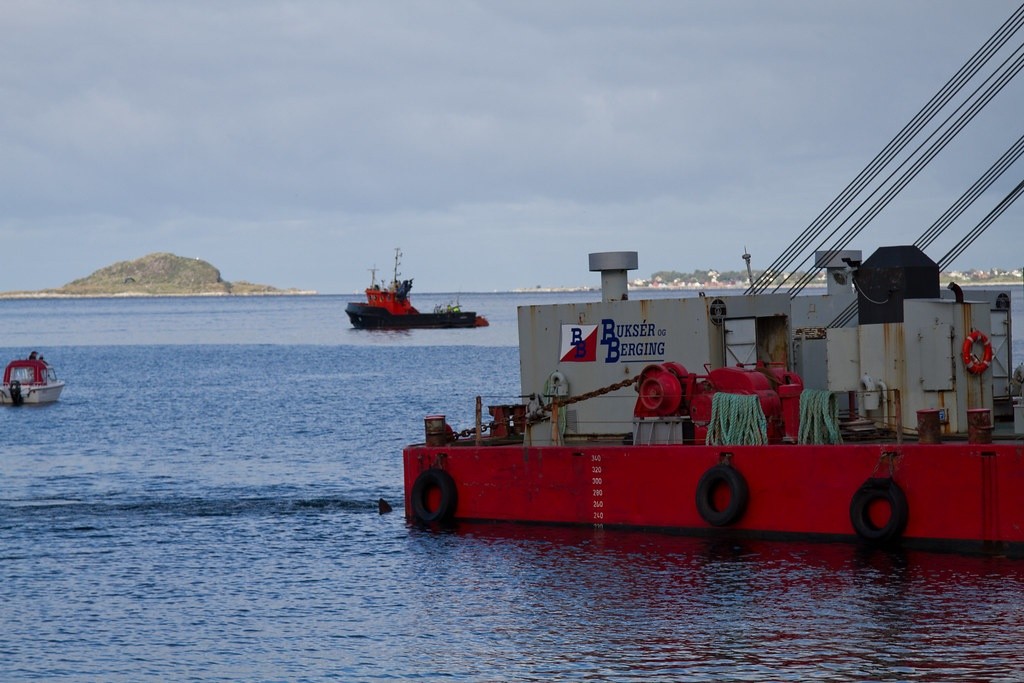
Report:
[28,350,44,361]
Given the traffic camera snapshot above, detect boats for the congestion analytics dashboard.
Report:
[344,246,489,332]
[401,5,1024,553]
[0,349,67,406]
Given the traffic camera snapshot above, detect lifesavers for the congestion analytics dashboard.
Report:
[961,330,993,374]
